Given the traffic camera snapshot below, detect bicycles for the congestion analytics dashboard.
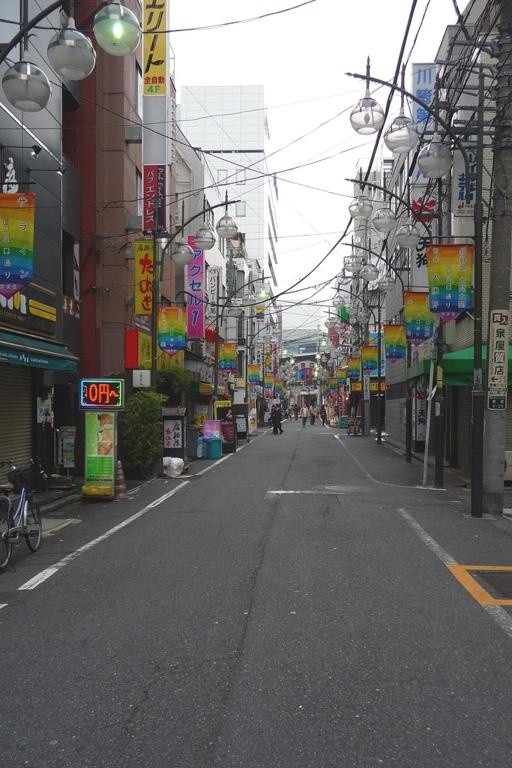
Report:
[0,457,47,571]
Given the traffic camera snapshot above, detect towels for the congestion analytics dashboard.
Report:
[339,416,349,428]
[203,438,224,460]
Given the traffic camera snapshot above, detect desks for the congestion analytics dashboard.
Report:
[151,199,294,395]
[0,0,141,112]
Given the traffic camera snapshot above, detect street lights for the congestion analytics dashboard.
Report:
[0,0,141,112]
[322,281,382,446]
[339,234,413,466]
[344,165,444,491]
[343,56,486,518]
[151,199,294,395]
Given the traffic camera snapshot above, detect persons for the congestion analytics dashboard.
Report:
[309,403,317,425]
[320,405,326,425]
[293,404,300,421]
[300,403,309,426]
[272,403,283,434]
[271,405,278,433]
